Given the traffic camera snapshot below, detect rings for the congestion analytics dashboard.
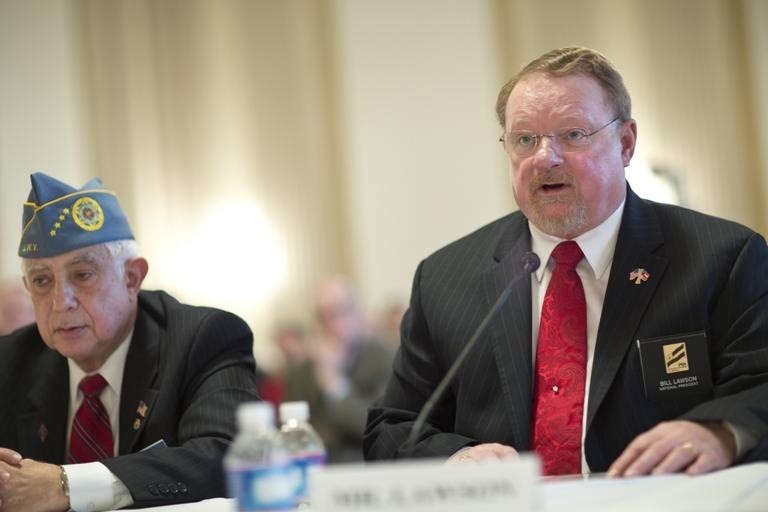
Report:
[680,441,700,454]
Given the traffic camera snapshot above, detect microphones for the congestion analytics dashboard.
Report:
[400,251,541,460]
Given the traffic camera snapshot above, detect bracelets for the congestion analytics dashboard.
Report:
[58,465,70,498]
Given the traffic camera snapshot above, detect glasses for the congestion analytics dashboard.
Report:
[499,115,619,159]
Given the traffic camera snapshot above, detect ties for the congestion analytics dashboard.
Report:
[69,375,113,465]
[531,241,587,476]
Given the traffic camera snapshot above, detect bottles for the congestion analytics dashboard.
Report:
[222,399,299,512]
[275,400,328,512]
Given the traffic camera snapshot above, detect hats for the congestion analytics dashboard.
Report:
[19,172,134,257]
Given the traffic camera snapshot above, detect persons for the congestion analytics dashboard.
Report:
[0,168,265,512]
[358,46,767,478]
[285,278,397,463]
